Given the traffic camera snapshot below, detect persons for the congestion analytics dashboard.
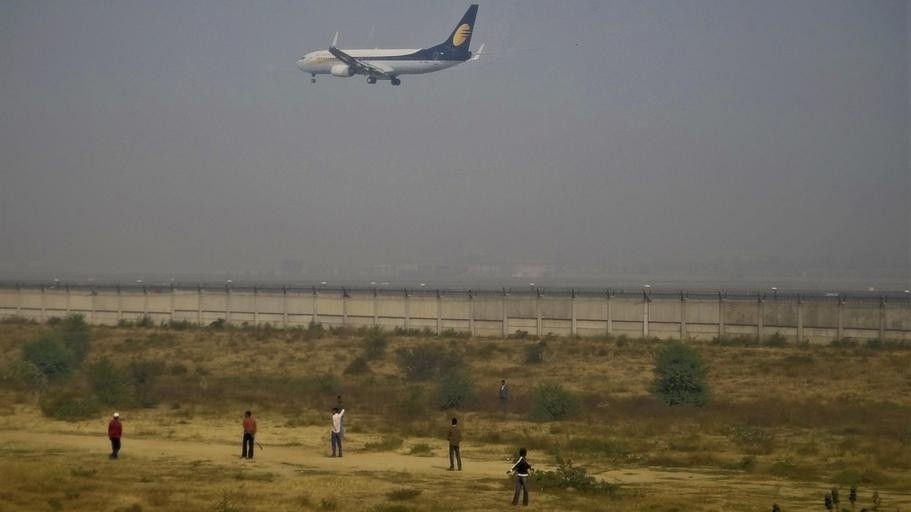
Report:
[447,418,463,471]
[336,395,346,442]
[499,379,509,412]
[239,410,258,459]
[506,447,537,507]
[106,410,123,460]
[329,406,347,457]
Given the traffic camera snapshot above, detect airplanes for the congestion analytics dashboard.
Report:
[294,3,488,87]
